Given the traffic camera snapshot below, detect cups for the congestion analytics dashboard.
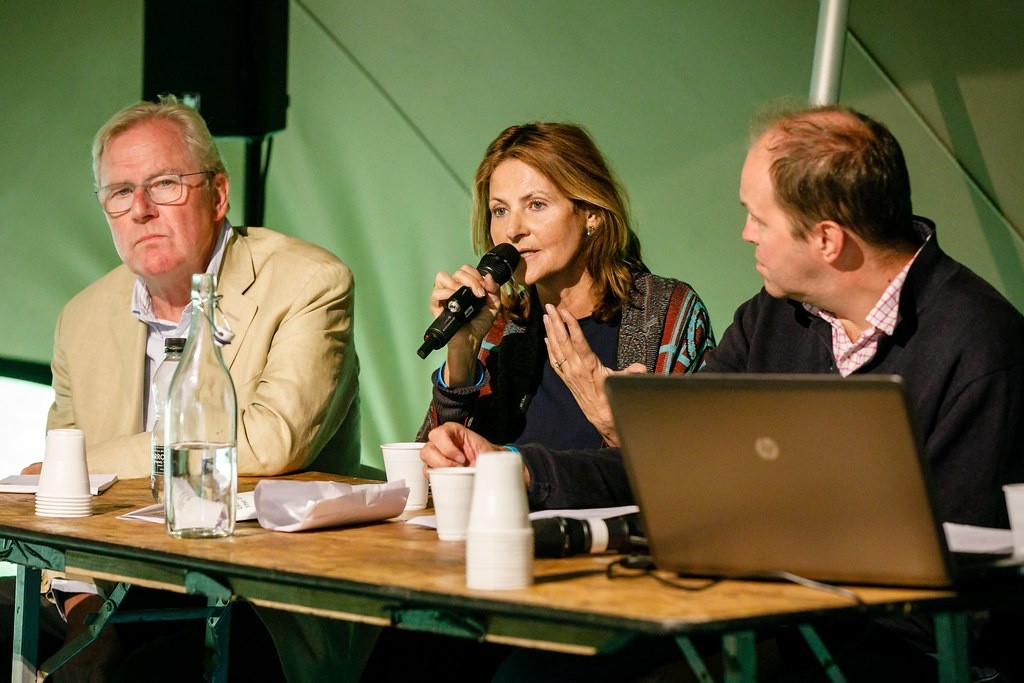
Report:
[36,429,93,517]
[466,451,535,590]
[380,442,429,510]
[1002,483,1024,557]
[427,466,475,541]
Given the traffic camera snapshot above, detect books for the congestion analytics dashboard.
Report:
[0,474,118,495]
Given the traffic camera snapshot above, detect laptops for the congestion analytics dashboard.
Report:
[602,373,1024,591]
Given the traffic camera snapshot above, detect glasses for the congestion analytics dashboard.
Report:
[95,171,216,213]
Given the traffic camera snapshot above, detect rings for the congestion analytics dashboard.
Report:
[556,359,566,369]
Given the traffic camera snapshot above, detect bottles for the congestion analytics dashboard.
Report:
[152,338,187,504]
[164,273,237,538]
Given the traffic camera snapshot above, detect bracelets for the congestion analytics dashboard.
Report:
[503,446,519,452]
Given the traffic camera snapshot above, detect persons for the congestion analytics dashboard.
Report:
[419,104,1024,683]
[359,123,716,683]
[0,101,360,683]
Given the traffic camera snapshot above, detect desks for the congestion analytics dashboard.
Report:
[0,476,980,683]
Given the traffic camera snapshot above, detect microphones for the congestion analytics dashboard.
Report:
[417,243,521,359]
[530,511,651,560]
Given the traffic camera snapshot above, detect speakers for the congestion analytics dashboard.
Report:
[142,0,290,137]
